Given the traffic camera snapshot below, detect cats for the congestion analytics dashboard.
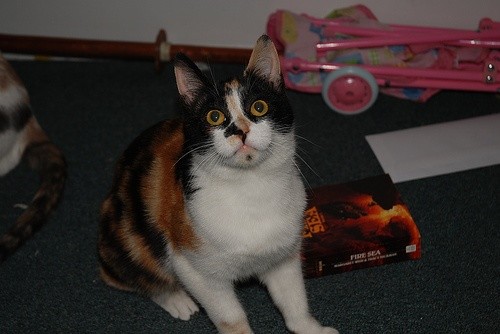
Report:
[96,33,337,334]
[0,52,66,266]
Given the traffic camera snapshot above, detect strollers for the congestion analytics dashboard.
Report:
[266,4,500,115]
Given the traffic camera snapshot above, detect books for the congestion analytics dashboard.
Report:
[301,173,422,278]
